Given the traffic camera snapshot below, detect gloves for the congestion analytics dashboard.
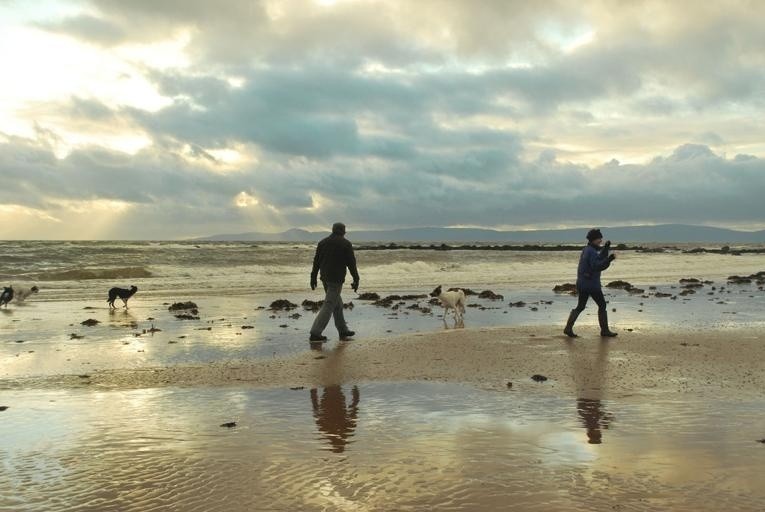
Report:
[609,253,616,262]
[310,272,318,291]
[351,276,360,293]
[605,240,612,249]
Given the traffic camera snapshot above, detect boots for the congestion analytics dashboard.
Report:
[597,310,618,337]
[563,308,579,339]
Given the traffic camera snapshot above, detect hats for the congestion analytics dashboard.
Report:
[587,228,602,241]
[331,223,346,235]
[587,434,601,444]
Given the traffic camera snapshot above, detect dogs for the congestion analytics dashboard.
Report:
[0,285,14,307]
[14,285,39,301]
[106,285,138,310]
[429,285,466,320]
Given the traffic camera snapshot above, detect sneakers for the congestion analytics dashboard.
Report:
[339,330,355,338]
[309,335,327,343]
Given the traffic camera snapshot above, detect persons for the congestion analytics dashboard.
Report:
[564,225,619,341]
[306,221,360,341]
